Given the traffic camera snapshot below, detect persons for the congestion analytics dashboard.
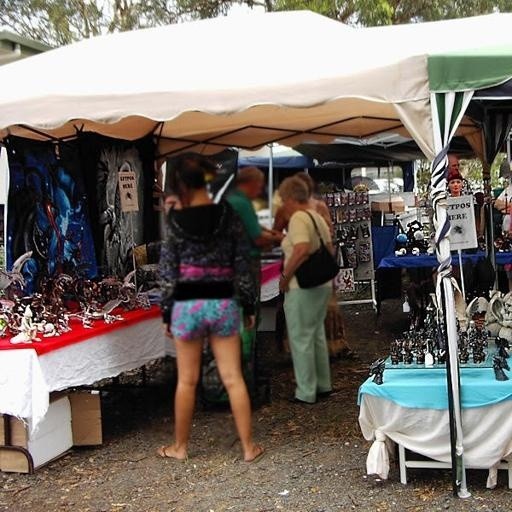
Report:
[446,154,512,238]
[225,167,340,404]
[156,158,266,462]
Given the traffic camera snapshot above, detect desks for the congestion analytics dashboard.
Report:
[0,300,163,476]
[360,347,512,494]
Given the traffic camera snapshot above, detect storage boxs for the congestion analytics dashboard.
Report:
[372,197,405,212]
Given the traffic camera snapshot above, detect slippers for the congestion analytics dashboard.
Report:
[244,444,266,464]
[155,444,188,462]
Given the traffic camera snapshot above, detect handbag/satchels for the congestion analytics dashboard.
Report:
[293,245,339,288]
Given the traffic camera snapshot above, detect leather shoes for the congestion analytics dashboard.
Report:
[287,391,331,405]
